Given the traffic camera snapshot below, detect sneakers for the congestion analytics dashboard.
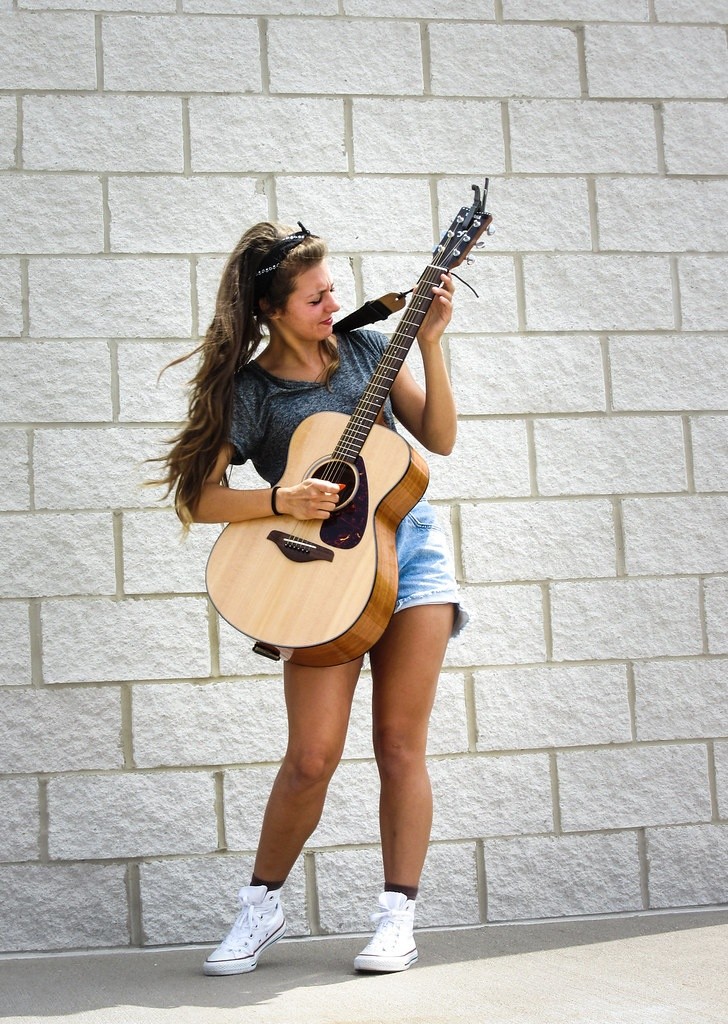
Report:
[202,885,287,976]
[355,892,419,972]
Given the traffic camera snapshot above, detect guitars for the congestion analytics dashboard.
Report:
[205,178,498,668]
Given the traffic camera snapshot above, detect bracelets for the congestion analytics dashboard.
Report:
[271,486,282,516]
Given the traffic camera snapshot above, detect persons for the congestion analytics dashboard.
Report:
[140,222,469,975]
